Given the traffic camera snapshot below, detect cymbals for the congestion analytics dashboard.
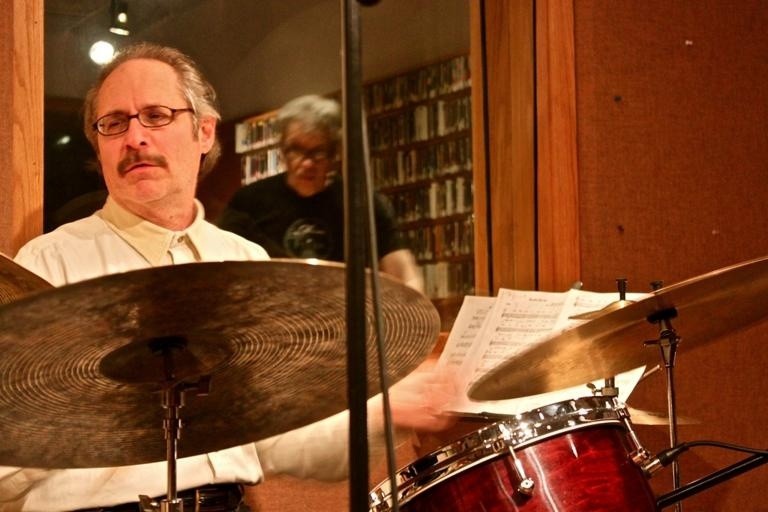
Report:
[468,257,768,401]
[0,260,441,467]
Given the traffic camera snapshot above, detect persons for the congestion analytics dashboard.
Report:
[212,95,423,297]
[0,43,466,511]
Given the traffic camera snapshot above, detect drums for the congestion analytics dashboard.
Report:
[369,396,662,512]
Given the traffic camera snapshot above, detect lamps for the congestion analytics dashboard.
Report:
[75,0,137,73]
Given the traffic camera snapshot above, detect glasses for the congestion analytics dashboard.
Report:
[276,143,337,167]
[91,104,195,136]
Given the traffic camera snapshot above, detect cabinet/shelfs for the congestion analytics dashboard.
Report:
[228,54,481,300]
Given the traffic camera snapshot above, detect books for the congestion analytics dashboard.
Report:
[364,56,475,299]
[237,114,288,185]
